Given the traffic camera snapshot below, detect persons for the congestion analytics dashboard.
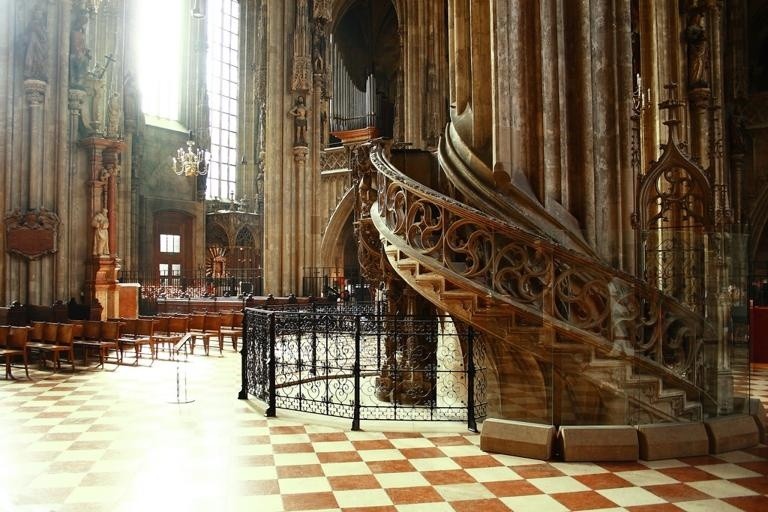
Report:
[744,278,764,309]
[90,206,110,254]
[289,95,312,148]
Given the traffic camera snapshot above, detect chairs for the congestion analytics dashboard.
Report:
[0,309,247,380]
